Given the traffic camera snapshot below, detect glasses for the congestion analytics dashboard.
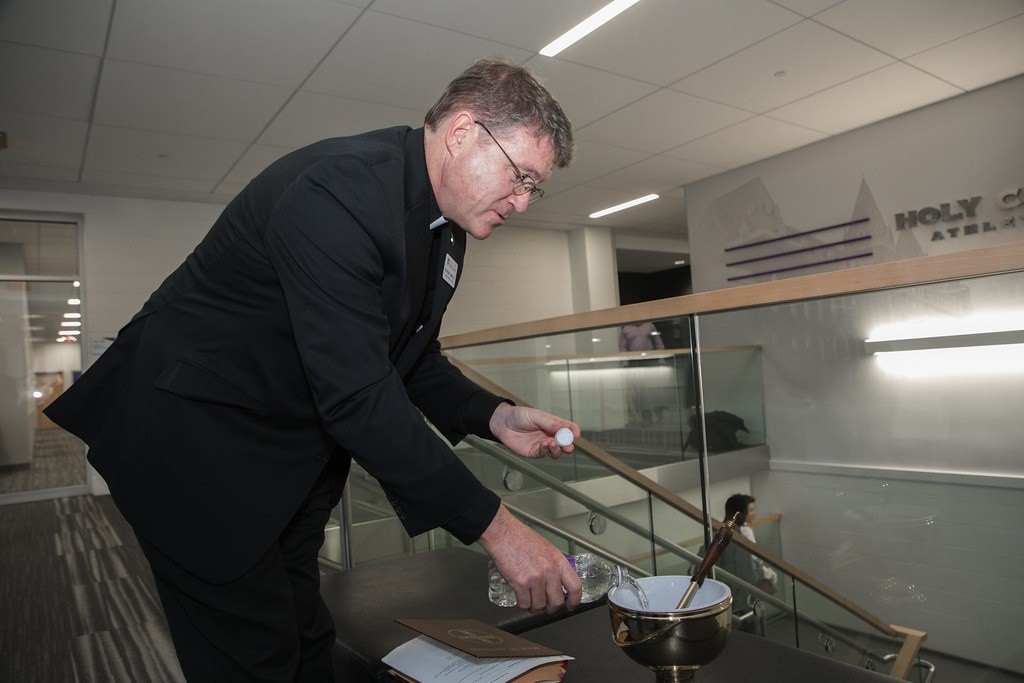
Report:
[474,120,545,205]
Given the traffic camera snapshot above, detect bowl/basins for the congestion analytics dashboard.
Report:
[603,575,735,674]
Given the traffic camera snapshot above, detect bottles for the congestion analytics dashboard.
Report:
[486,555,631,611]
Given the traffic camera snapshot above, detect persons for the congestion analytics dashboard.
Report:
[699,493,777,635]
[40,58,583,682]
[618,321,667,429]
